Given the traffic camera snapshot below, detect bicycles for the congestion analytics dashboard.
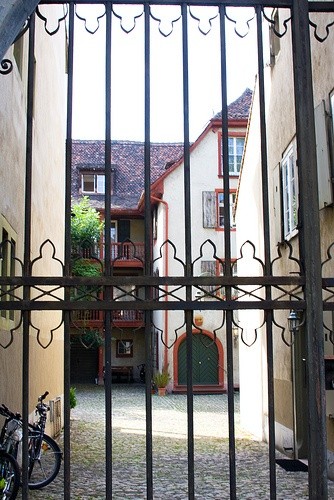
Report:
[14,391,62,489]
[0,403,34,500]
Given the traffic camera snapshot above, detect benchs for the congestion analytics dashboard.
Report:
[103,365,134,383]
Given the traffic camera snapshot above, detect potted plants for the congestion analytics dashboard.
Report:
[154,370,171,396]
